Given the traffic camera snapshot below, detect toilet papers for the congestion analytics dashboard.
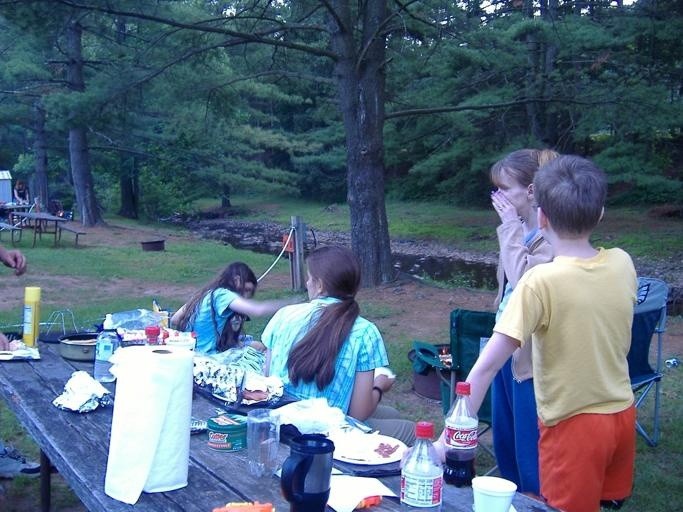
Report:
[99,344,196,506]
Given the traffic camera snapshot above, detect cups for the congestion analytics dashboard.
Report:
[472,476,517,512]
[247,408,280,478]
[281,436,335,512]
[166,336,196,352]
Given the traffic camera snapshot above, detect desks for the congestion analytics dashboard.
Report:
[0,342,559,512]
[11,212,67,248]
[3,205,32,227]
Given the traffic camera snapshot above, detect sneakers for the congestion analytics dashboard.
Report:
[0,444,42,479]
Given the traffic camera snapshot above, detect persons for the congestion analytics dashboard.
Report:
[489,149,556,501]
[1,243,41,481]
[171,262,307,377]
[13,180,29,226]
[432,155,638,511]
[261,244,417,449]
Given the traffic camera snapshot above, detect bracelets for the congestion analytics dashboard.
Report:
[373,386,382,402]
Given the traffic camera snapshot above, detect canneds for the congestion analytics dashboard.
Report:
[206,413,247,453]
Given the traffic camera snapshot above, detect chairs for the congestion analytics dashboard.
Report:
[412,308,500,476]
[626,277,668,447]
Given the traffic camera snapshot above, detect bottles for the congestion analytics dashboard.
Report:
[145,327,160,345]
[400,421,443,512]
[444,381,480,487]
[94,314,118,382]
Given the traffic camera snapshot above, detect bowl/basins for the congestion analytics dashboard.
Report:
[57,332,97,361]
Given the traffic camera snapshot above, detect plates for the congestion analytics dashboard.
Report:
[331,434,407,466]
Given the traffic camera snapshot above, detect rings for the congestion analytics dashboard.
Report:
[498,206,503,210]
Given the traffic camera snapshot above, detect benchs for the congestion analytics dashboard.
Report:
[57,224,87,246]
[0,223,22,248]
